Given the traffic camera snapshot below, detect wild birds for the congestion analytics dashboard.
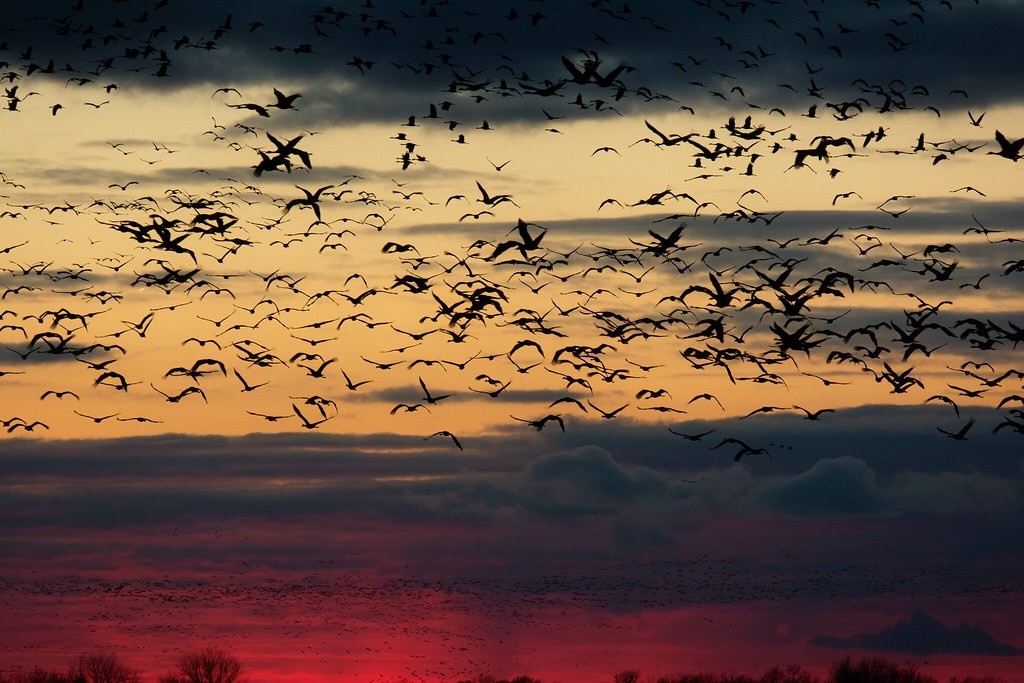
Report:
[0,0,1024,465]
